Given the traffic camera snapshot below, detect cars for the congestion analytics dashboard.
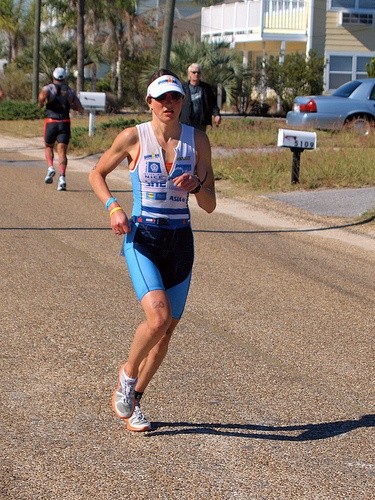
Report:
[285,77,375,139]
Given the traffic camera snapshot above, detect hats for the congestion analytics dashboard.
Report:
[147,75,187,99]
[52,67,66,80]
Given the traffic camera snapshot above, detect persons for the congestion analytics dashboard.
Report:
[88,69,217,431]
[37,67,83,191]
[180,64,222,133]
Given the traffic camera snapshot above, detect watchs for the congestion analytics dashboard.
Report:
[190,182,202,194]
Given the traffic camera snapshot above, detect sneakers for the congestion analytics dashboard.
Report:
[122,405,152,432]
[45,166,56,184]
[111,361,139,420]
[56,179,66,191]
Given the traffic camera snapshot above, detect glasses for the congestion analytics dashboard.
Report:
[154,93,183,102]
[191,71,203,75]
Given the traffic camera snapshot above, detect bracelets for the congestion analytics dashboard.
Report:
[106,197,123,216]
